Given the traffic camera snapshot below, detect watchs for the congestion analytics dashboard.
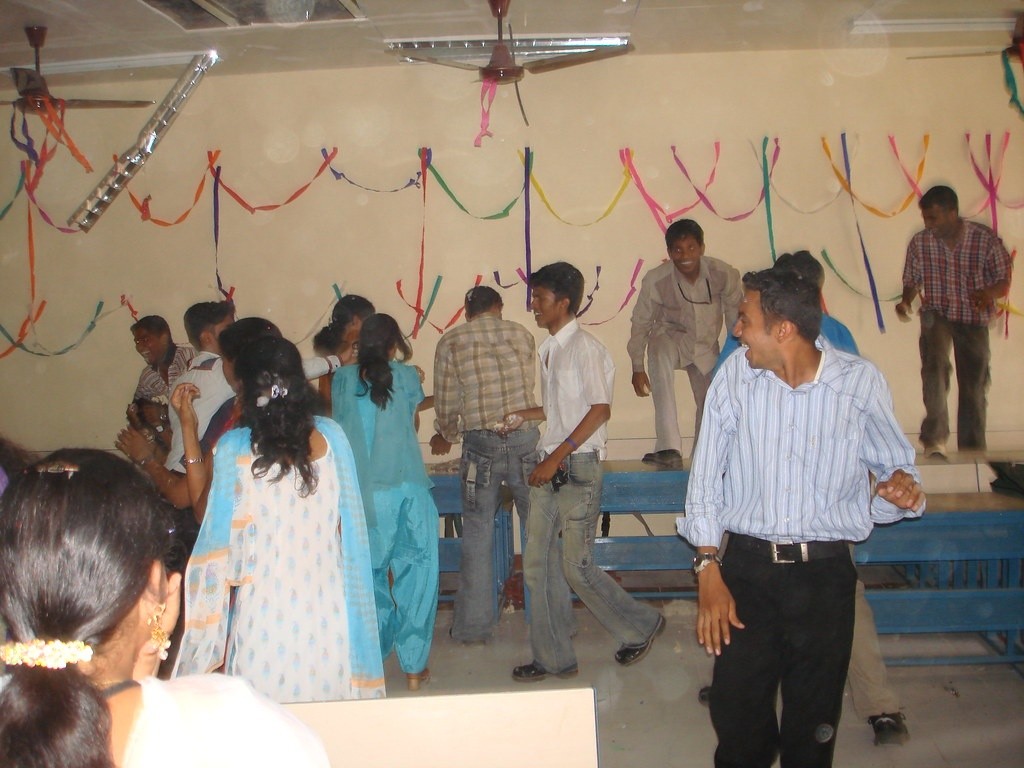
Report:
[693,552,723,573]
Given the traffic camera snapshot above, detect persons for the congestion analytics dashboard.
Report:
[0,447,332,768]
[697,251,910,747]
[429,284,579,644]
[162,301,357,473]
[172,336,386,704]
[313,295,440,691]
[895,185,1012,455]
[626,220,743,464]
[483,262,667,682]
[113,315,283,556]
[675,269,926,767]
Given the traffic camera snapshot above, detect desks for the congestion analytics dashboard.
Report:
[283,687,601,768]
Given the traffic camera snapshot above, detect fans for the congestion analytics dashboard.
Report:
[384,0,636,86]
[0,24,156,116]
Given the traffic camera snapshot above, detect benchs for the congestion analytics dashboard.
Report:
[852,511,1023,667]
[427,471,698,603]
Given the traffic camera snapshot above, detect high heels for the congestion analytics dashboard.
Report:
[408,669,428,691]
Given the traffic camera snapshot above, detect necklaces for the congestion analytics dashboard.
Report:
[103,679,140,697]
[678,279,712,304]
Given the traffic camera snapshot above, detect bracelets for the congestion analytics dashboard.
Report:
[183,457,204,465]
[565,438,576,450]
[139,454,152,467]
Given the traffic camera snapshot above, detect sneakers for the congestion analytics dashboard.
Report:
[699,688,711,704]
[871,713,911,745]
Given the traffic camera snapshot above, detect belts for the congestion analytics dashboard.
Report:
[728,533,845,564]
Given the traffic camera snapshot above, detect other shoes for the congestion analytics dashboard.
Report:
[615,613,666,665]
[512,663,578,683]
[923,444,947,459]
